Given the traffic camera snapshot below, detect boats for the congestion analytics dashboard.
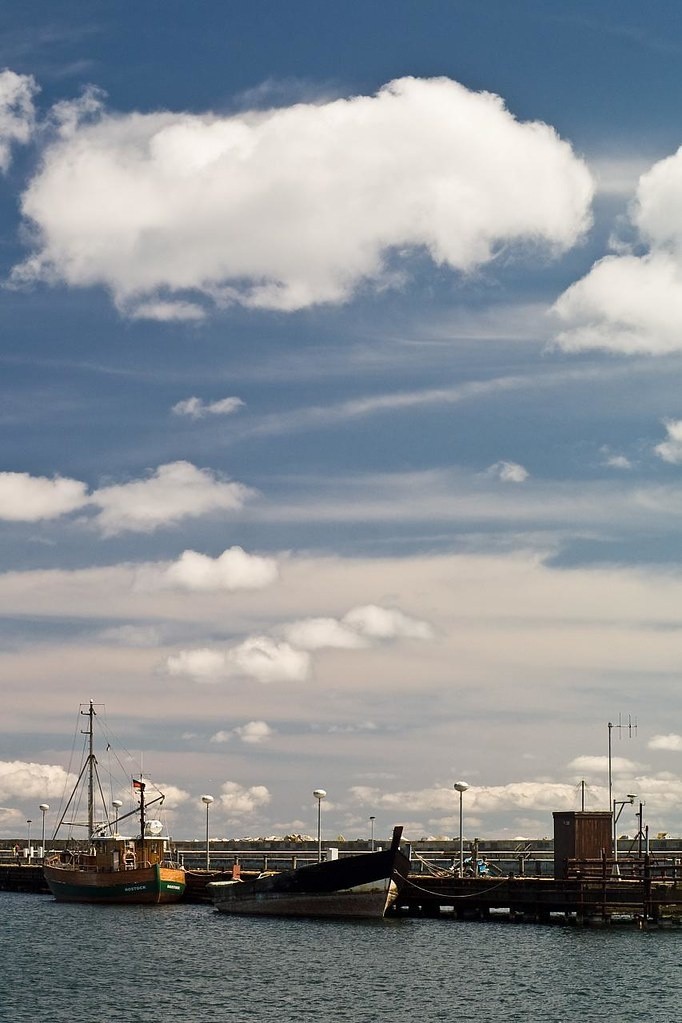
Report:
[201,826,412,919]
[42,699,186,904]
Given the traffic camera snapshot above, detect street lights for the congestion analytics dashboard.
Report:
[453,780,470,878]
[369,815,376,851]
[201,795,215,872]
[39,803,50,850]
[313,788,328,863]
[26,820,32,848]
[111,799,122,834]
